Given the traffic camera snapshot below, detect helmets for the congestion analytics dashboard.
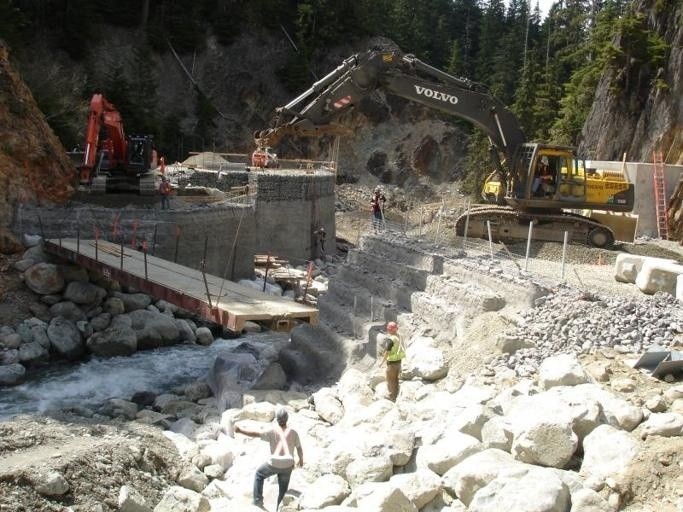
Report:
[388,321,398,334]
[162,176,167,180]
[276,409,289,424]
[375,187,381,194]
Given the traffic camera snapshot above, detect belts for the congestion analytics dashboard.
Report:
[388,359,402,366]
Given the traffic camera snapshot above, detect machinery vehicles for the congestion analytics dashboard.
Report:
[78,94,154,196]
[254,47,637,249]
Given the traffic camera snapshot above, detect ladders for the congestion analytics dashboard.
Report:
[652,151,668,241]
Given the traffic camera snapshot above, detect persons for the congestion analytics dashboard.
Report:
[159,154,165,176]
[159,176,171,210]
[369,188,386,234]
[234,411,302,511]
[377,323,405,402]
[318,227,328,256]
[532,160,556,195]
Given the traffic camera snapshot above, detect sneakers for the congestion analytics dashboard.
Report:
[385,396,394,402]
